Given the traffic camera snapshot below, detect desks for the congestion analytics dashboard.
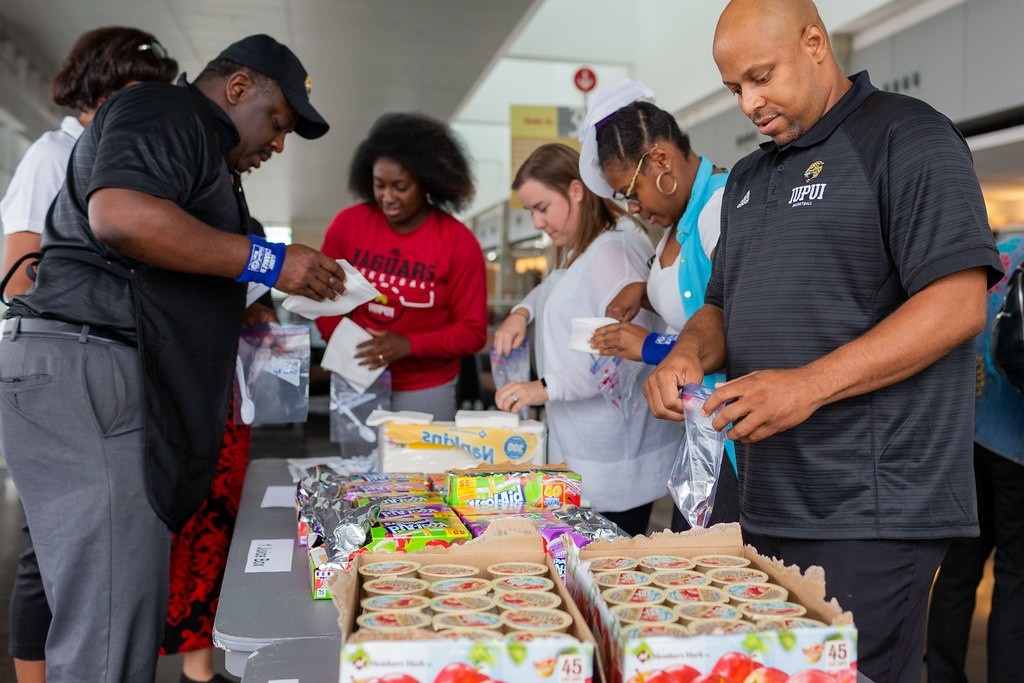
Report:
[212,460,342,683]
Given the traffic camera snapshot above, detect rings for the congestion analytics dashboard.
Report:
[510,392,517,402]
[378,354,384,362]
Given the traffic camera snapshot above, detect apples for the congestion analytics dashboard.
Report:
[624,652,858,683]
[347,662,507,683]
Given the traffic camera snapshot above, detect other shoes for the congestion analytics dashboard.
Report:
[180,672,233,683]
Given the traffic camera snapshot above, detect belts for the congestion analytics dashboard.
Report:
[2,317,138,349]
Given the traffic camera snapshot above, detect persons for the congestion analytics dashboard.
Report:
[639,0,1005,683]
[318,110,491,426]
[491,144,671,535]
[579,81,742,528]
[0,27,175,682]
[0,33,347,682]
[924,233,1024,683]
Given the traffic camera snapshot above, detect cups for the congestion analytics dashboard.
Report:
[357,554,829,644]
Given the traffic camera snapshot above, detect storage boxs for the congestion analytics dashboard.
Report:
[325,516,858,683]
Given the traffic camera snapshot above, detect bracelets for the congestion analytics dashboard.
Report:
[641,330,678,366]
[511,312,528,321]
[540,377,550,401]
[239,234,286,287]
[248,301,264,315]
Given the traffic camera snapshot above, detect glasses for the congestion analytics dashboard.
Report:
[136,40,168,61]
[613,151,649,204]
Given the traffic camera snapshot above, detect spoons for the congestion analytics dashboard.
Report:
[236,355,255,425]
[341,406,377,443]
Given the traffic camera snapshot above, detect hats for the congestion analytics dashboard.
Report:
[218,34,330,139]
